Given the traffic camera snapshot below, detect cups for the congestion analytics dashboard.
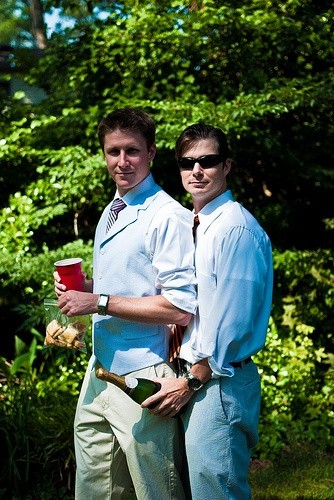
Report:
[54,258,83,293]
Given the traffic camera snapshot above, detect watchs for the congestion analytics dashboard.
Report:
[184,371,203,390]
[97,293,109,315]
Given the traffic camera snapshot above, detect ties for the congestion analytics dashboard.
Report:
[105,198,127,235]
[191,216,200,243]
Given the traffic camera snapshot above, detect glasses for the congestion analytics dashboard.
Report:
[178,154,224,168]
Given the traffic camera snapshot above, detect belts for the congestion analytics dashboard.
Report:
[175,356,252,374]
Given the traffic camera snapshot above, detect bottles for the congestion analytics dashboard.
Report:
[94,368,185,419]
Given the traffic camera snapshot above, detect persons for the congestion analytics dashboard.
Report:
[142,123,271,500]
[54,106,196,500]
